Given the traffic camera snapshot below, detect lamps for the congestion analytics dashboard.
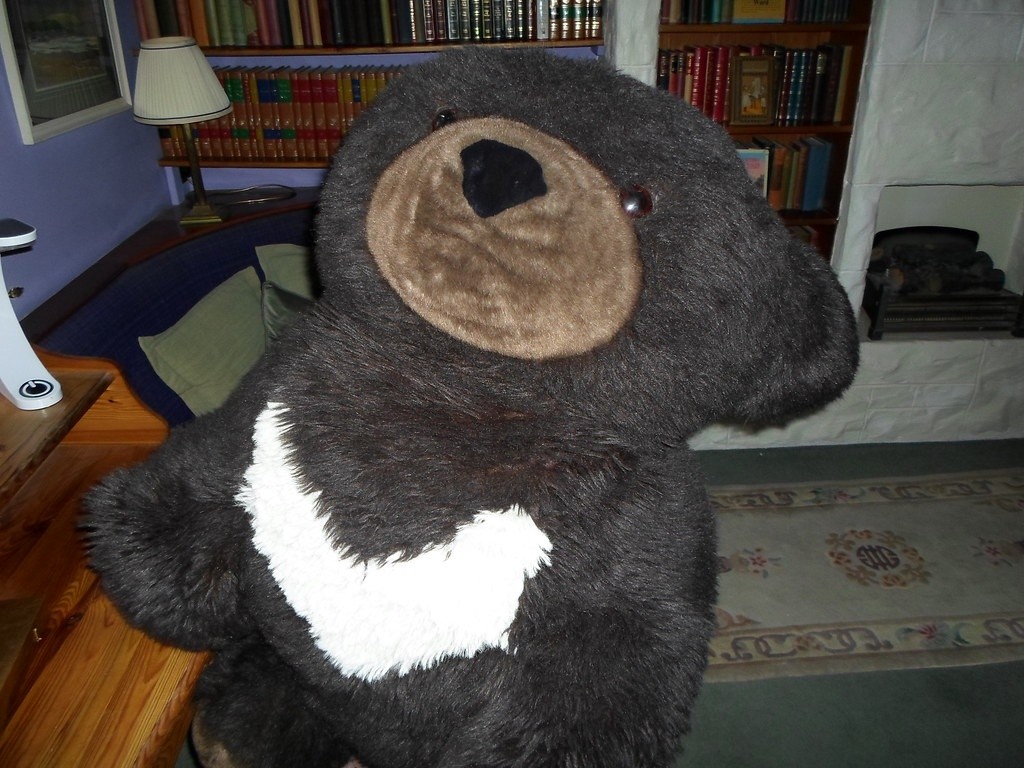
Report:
[133,36,226,223]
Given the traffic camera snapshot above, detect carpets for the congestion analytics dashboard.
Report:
[703,467,1024,682]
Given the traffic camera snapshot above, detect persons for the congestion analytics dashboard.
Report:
[742,80,768,112]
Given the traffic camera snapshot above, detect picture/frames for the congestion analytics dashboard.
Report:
[0,0,133,145]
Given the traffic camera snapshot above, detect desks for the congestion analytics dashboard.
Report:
[0,347,219,768]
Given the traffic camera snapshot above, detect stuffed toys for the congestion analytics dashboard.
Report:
[874,244,1005,293]
[73,46,863,768]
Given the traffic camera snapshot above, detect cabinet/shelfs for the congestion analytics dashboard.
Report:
[658,0,873,268]
[159,38,603,169]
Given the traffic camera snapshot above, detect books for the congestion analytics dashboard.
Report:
[731,135,849,210]
[656,43,750,125]
[157,64,411,158]
[660,0,851,24]
[751,47,853,121]
[132,0,603,47]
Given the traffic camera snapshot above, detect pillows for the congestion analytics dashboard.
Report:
[138,266,262,416]
[255,244,312,299]
[261,280,312,353]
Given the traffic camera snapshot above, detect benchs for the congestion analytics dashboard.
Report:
[38,207,321,428]
